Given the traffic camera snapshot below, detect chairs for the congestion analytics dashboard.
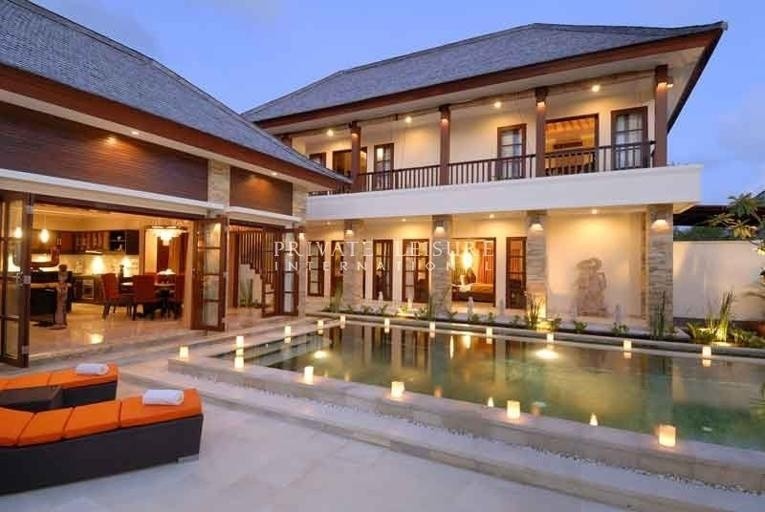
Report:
[99,272,184,320]
[0,361,202,496]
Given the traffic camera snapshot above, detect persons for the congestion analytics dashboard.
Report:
[57,263,73,313]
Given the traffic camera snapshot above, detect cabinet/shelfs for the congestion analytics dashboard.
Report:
[12,227,139,256]
